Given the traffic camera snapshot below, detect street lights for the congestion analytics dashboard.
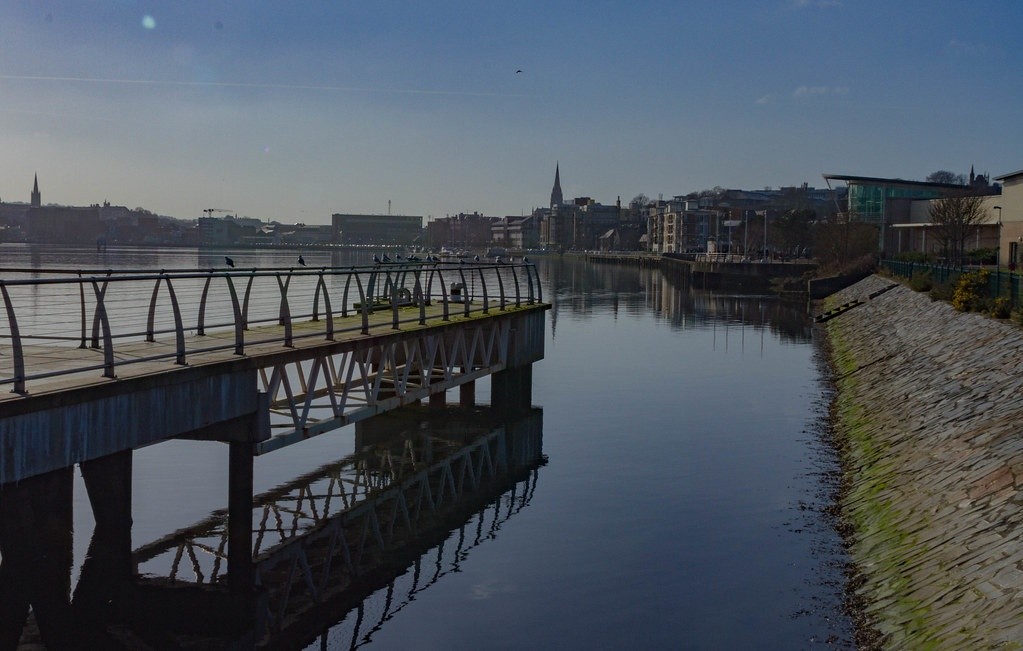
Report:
[993,206,1002,271]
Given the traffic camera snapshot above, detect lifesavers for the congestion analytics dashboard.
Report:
[396,288,411,304]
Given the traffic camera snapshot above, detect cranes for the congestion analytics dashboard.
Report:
[203,208,234,217]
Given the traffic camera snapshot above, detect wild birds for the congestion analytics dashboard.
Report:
[473,254,531,266]
[373,252,440,263]
[224,256,235,269]
[297,254,307,266]
